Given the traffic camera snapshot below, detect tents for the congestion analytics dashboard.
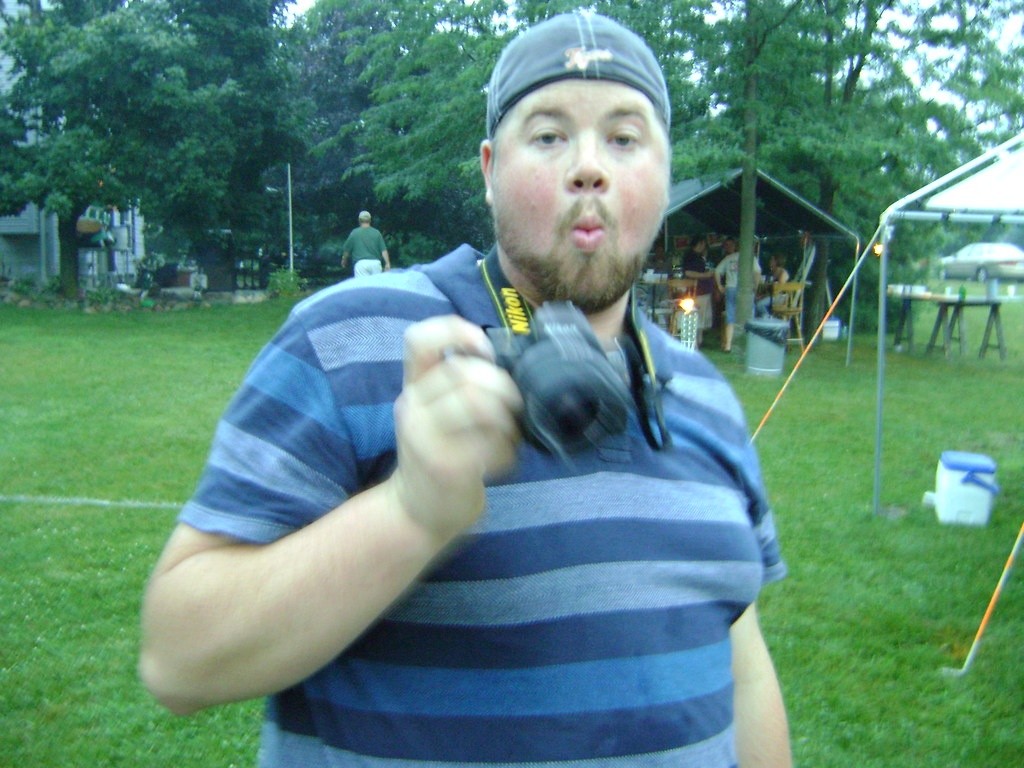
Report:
[658,165,856,367]
[874,125,1024,517]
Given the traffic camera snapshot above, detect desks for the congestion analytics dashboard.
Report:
[887,290,1018,362]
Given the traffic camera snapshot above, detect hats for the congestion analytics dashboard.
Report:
[359,211,371,219]
[487,13,671,141]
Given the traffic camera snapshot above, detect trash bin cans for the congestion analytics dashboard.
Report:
[743,318,790,376]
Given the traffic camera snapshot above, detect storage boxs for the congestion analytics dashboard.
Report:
[935,451,996,525]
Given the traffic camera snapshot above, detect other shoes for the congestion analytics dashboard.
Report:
[723,347,732,353]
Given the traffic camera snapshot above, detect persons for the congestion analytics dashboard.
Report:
[134,10,793,768]
[647,241,672,280]
[341,210,391,278]
[684,235,790,353]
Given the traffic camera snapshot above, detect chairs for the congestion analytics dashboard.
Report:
[668,279,703,346]
[770,282,806,354]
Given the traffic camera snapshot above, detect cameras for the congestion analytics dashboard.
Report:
[484,301,629,455]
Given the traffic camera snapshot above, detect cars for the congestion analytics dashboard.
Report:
[935,242,1024,282]
